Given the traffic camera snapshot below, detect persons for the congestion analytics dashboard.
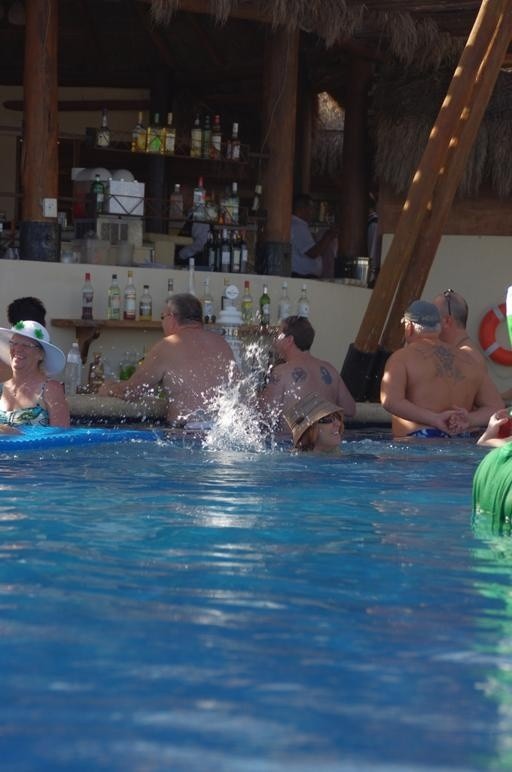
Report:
[242,316,358,439]
[379,300,478,447]
[8,298,47,328]
[284,393,348,456]
[473,442,511,534]
[282,192,337,279]
[431,288,503,435]
[362,189,382,287]
[1,319,74,433]
[99,292,242,435]
[174,177,212,267]
[474,407,512,446]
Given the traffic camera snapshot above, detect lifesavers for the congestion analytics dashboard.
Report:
[479,303,512,366]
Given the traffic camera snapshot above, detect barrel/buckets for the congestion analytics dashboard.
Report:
[19,221,62,262]
[333,256,371,283]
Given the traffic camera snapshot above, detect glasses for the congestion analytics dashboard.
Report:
[318,413,340,424]
[8,339,40,350]
[444,288,454,315]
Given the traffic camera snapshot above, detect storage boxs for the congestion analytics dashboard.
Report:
[73,178,146,217]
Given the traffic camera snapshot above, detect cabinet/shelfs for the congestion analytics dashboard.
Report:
[74,121,265,267]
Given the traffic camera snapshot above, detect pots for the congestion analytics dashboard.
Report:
[344,255,380,287]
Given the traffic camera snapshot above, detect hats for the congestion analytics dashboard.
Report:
[283,391,345,448]
[400,300,441,326]
[0,320,66,376]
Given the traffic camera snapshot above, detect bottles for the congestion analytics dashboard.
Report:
[89,108,267,274]
[139,284,152,320]
[199,278,216,324]
[123,270,137,321]
[244,344,288,395]
[166,278,175,297]
[80,271,94,319]
[107,274,121,321]
[219,276,312,325]
[61,341,146,395]
[186,257,197,299]
